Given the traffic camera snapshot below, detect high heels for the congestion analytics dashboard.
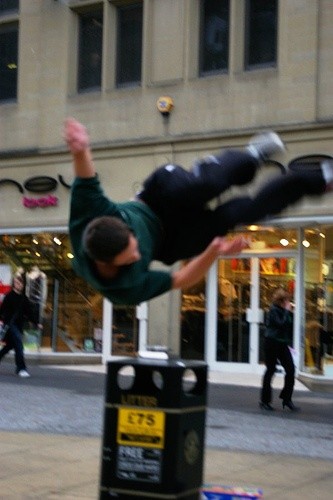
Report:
[260,400,275,411]
[283,399,300,412]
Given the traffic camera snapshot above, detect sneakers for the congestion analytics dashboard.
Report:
[319,161,333,185]
[246,132,286,162]
[17,369,30,377]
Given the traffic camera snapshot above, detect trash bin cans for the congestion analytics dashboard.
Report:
[95,355,210,499]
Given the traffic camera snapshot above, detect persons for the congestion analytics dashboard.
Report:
[62,115,332,305]
[259,293,301,414]
[0,271,44,378]
